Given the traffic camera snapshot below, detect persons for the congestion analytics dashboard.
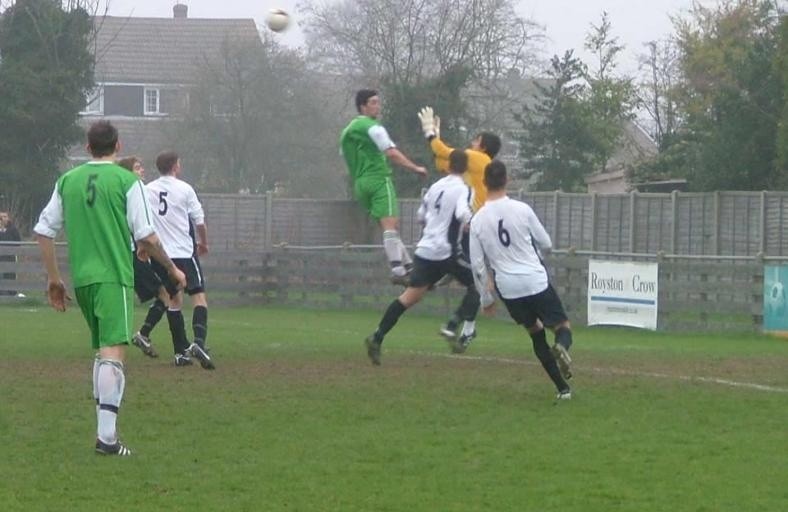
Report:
[418,106,501,337]
[468,161,574,399]
[364,150,479,364]
[340,88,426,285]
[0,209,23,302]
[119,153,214,370]
[35,120,188,455]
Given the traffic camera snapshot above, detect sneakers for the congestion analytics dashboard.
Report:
[429,273,452,290]
[96,438,133,455]
[132,331,157,357]
[391,271,412,286]
[453,329,476,353]
[367,335,381,364]
[550,343,573,379]
[439,327,455,337]
[175,343,215,370]
[556,390,571,401]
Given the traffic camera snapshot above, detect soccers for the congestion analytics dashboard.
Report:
[266,9,290,32]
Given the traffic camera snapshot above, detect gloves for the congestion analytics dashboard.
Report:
[418,106,442,140]
